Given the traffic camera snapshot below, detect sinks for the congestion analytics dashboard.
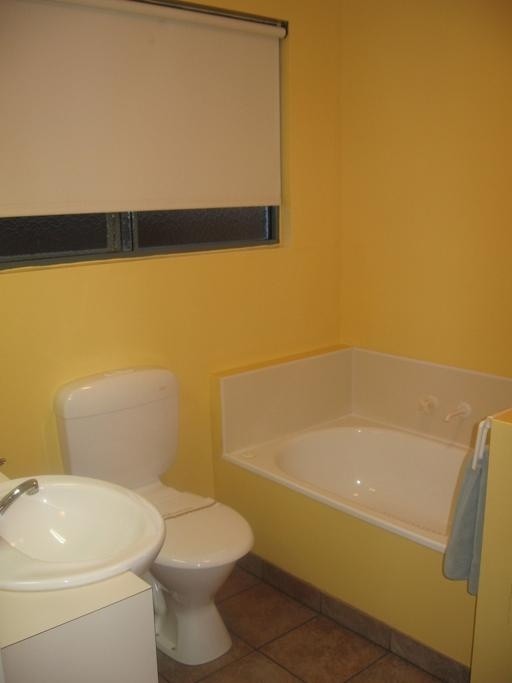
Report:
[0,474,166,593]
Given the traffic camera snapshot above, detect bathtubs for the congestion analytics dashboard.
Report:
[223,413,476,556]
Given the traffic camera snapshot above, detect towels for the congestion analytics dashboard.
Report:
[443,446,489,596]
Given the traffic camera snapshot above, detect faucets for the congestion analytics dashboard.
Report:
[444,407,465,422]
[1,478,40,515]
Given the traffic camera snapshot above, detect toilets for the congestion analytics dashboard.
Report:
[53,366,254,665]
